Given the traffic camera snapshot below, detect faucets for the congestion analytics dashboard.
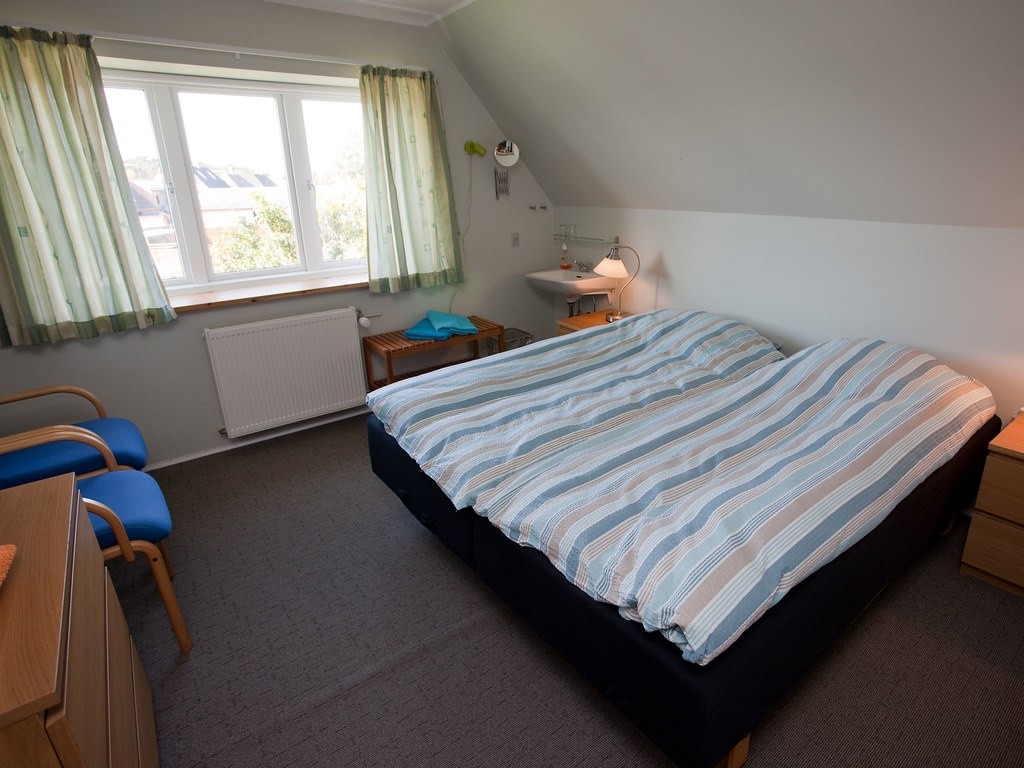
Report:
[572,259,592,271]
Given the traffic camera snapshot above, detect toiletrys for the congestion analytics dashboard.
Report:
[559,243,572,269]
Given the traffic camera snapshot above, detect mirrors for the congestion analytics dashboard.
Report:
[494,140,520,167]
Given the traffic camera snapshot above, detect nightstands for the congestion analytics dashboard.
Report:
[959,411,1024,597]
[555,307,634,337]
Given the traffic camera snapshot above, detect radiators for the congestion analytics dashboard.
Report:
[201,304,368,440]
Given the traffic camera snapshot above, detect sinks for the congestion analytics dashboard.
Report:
[524,267,616,295]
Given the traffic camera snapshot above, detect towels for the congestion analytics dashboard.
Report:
[402,310,480,341]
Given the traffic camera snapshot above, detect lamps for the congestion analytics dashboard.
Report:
[593,245,640,323]
[464,140,486,157]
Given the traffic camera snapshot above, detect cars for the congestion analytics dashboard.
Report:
[125,156,288,284]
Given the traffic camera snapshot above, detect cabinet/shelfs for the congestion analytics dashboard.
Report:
[0,472,162,768]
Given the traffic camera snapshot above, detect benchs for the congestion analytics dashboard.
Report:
[363,316,504,392]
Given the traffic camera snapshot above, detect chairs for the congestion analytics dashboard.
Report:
[1,385,194,655]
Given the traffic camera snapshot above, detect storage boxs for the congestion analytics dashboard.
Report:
[486,326,534,356]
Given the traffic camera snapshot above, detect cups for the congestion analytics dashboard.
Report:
[559,224,568,240]
[568,224,576,240]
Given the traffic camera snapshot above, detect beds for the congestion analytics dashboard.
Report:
[366,307,1007,767]
